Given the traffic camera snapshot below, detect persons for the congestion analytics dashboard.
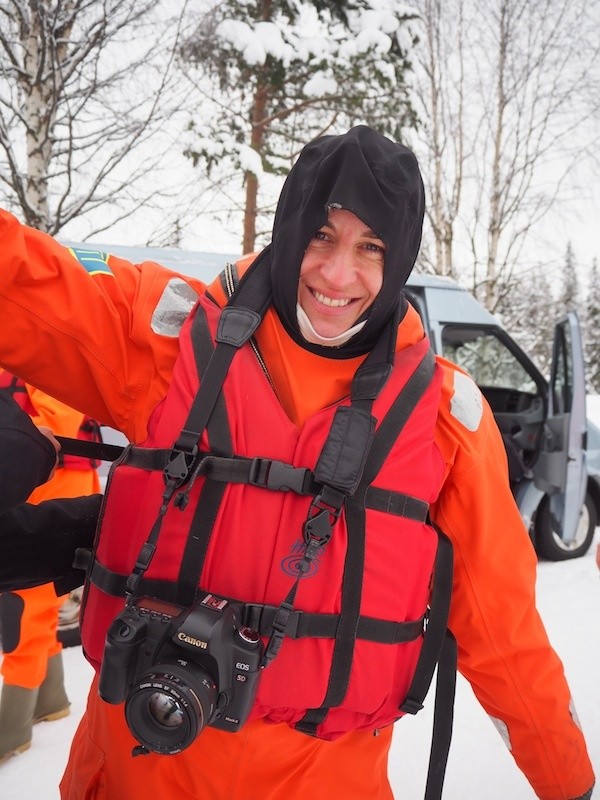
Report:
[0,366,102,765]
[0,127,596,800]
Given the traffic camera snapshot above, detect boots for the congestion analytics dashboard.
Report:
[33,653,72,723]
[0,684,38,765]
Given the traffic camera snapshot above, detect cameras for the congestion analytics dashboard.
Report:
[99,592,266,755]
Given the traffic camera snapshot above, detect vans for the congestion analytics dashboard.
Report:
[52,240,600,648]
